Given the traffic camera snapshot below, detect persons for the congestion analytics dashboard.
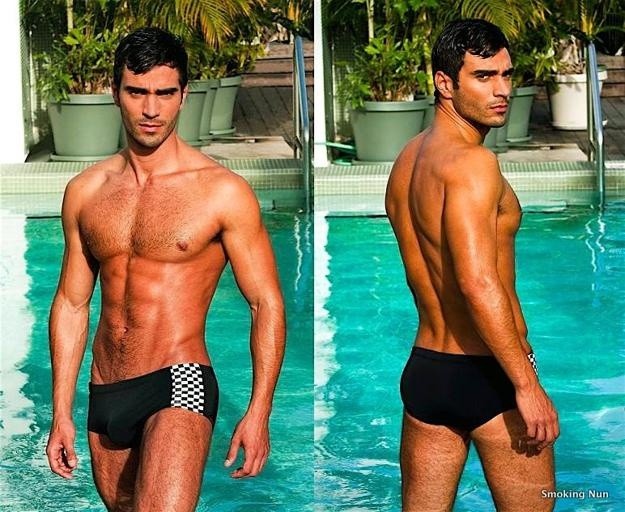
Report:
[42,25,288,512]
[384,17,557,512]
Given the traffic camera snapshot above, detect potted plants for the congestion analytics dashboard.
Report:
[320,0,613,172]
[23,1,314,163]
